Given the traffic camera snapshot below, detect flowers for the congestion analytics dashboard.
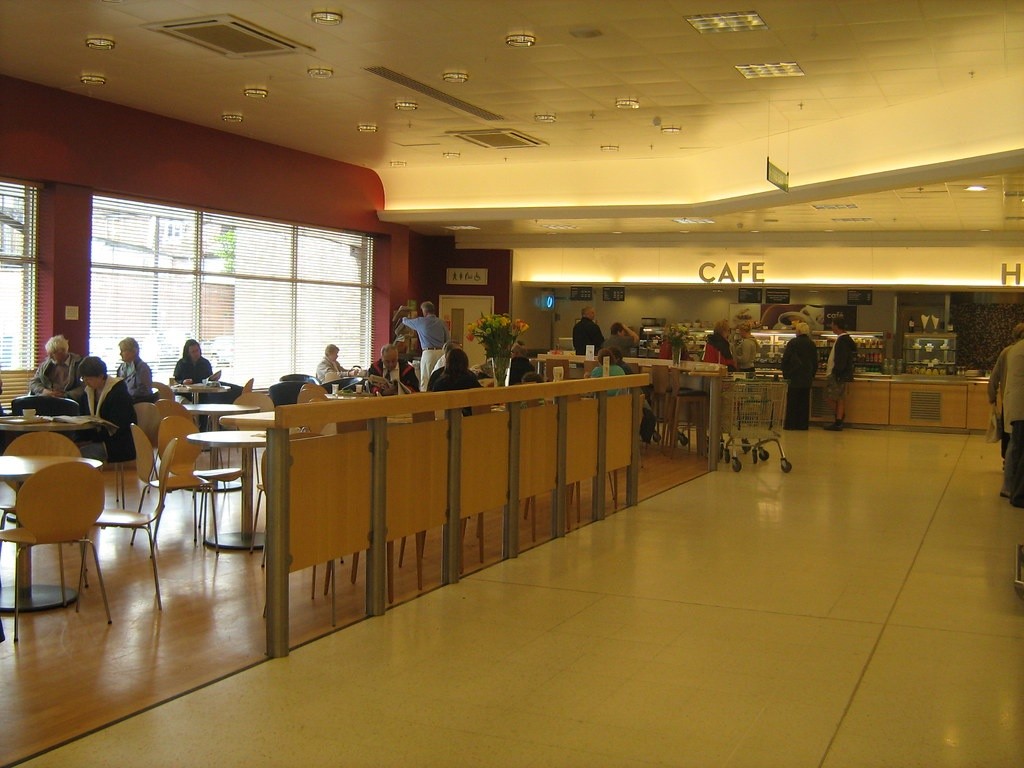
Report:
[465,311,531,386]
[660,320,692,363]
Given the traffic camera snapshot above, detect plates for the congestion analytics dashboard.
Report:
[964,370,981,376]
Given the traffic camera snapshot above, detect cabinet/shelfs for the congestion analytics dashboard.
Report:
[903,332,956,375]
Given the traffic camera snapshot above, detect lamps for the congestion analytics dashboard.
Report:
[615,97,638,109]
[534,112,557,123]
[395,99,418,112]
[443,151,461,160]
[311,7,343,26]
[85,34,115,51]
[307,66,333,80]
[222,112,243,123]
[357,123,378,133]
[80,72,106,86]
[243,88,269,98]
[660,124,683,136]
[442,68,469,83]
[505,33,536,47]
[600,144,619,153]
[390,160,407,168]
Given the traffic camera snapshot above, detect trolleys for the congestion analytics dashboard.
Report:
[649,371,793,475]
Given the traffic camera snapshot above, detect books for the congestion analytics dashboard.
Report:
[370,374,412,394]
[53,415,119,437]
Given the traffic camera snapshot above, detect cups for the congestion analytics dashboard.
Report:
[331,384,339,395]
[355,385,363,394]
[23,409,36,418]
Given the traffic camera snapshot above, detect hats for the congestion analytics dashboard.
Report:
[1014,324,1024,337]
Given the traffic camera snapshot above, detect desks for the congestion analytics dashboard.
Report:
[0,455,105,613]
[170,385,231,451]
[219,411,276,429]
[183,404,261,493]
[0,415,96,433]
[324,394,375,401]
[185,431,267,550]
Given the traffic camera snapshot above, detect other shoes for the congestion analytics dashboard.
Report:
[824,421,843,431]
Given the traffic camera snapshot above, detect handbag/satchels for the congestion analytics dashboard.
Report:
[12,395,78,416]
[985,403,1001,442]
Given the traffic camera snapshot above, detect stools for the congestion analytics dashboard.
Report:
[584,360,599,377]
[652,364,693,448]
[625,362,660,444]
[665,367,707,460]
[545,358,571,383]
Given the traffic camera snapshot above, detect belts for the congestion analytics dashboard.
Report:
[423,348,437,350]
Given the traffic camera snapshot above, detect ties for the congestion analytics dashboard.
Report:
[385,370,390,381]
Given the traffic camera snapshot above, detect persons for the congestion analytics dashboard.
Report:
[509,343,535,386]
[572,305,605,356]
[519,371,545,410]
[733,321,757,426]
[402,301,451,393]
[117,337,152,403]
[823,317,856,431]
[703,320,737,372]
[781,322,818,430]
[584,347,646,396]
[427,340,484,417]
[317,344,360,384]
[659,319,692,361]
[29,334,88,402]
[75,356,137,472]
[986,322,1024,508]
[601,322,639,357]
[173,339,213,433]
[367,344,420,396]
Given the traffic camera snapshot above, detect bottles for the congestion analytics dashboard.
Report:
[956,365,967,376]
[850,337,903,375]
[814,349,830,374]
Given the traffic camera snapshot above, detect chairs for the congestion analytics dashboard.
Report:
[0,375,331,643]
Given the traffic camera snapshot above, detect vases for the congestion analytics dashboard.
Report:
[492,357,512,406]
[671,345,681,364]
